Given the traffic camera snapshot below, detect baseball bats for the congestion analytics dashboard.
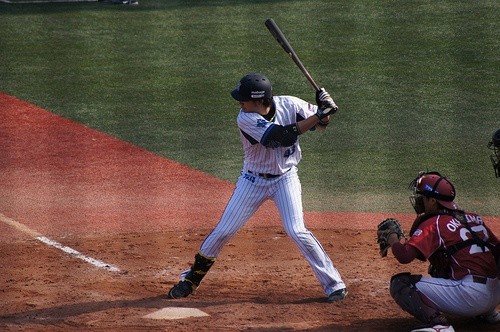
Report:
[263,17,338,114]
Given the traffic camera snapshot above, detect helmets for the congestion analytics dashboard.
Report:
[229,74,274,101]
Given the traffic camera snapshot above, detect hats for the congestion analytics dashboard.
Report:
[416,171,459,211]
[493,129,500,146]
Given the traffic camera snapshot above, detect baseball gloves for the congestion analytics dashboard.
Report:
[376,216,407,259]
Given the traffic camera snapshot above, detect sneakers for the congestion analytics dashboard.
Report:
[328,288,349,301]
[169,276,201,300]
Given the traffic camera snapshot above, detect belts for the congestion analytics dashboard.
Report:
[247,170,280,178]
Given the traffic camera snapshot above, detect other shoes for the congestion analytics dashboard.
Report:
[485,311,500,324]
[410,324,455,332]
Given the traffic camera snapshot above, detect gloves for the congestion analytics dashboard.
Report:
[315,87,338,121]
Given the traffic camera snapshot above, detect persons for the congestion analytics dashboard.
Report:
[389,171,500,331]
[169,74,348,301]
